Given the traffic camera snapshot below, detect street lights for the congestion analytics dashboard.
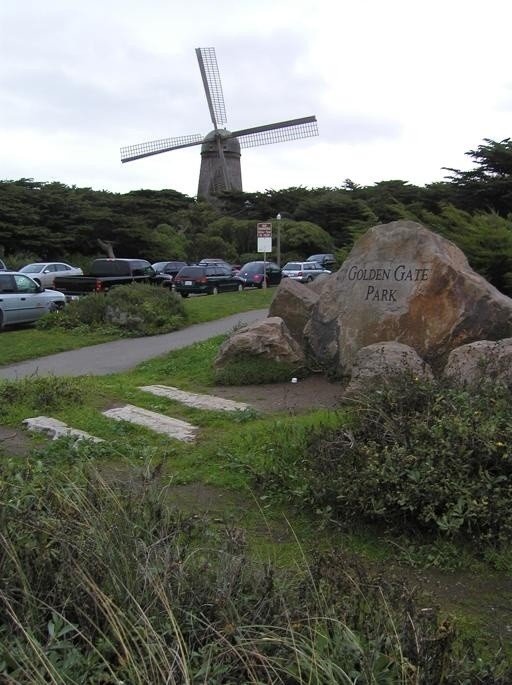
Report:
[276,213,281,266]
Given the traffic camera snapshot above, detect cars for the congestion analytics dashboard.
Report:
[151,253,336,298]
[0,258,83,332]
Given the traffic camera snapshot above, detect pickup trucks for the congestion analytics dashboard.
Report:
[55,258,173,297]
